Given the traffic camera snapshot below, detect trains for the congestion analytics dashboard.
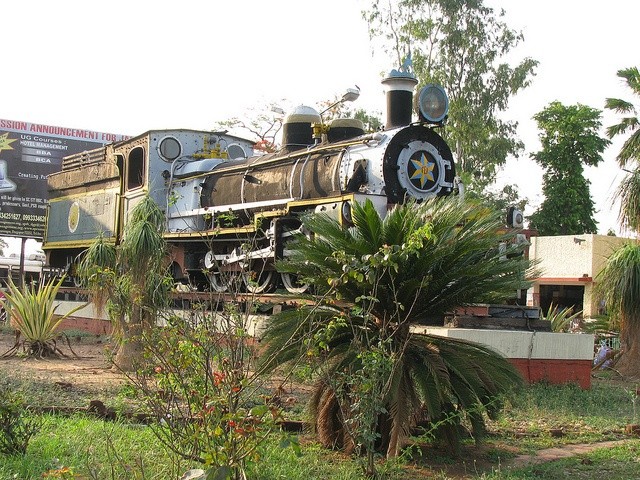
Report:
[39,57,532,299]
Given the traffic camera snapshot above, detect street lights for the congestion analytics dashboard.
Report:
[318,82,361,117]
